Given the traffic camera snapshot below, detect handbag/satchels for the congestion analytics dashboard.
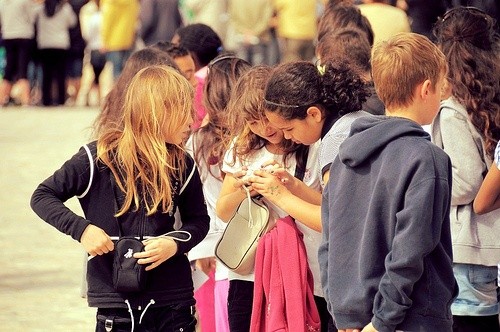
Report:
[214,198,274,276]
[113,238,145,291]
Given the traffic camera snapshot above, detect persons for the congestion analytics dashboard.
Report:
[218,64,322,332]
[314,2,499,332]
[30,24,250,332]
[265,58,374,332]
[0,0,500,108]
